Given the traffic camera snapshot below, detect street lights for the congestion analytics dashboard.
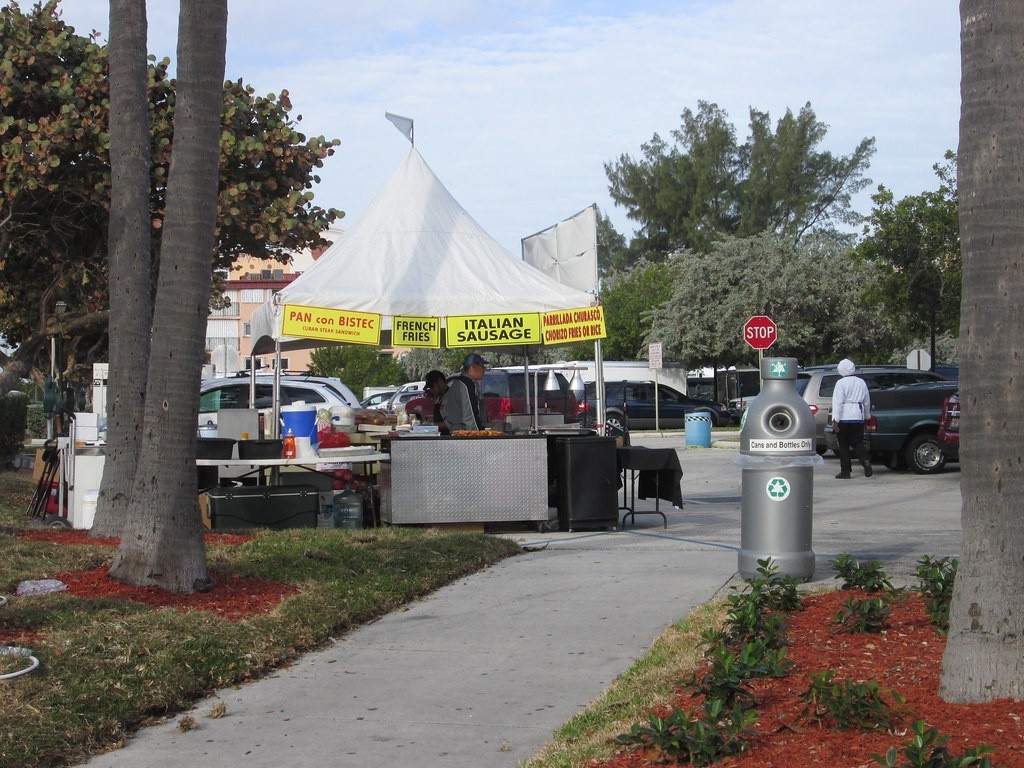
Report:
[54,300,67,436]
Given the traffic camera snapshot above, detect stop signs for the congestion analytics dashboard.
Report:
[743,315,778,349]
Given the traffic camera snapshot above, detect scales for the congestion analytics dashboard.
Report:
[534,366,591,436]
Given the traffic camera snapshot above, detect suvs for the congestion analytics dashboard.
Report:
[796,361,960,475]
[192,363,585,439]
[578,380,731,433]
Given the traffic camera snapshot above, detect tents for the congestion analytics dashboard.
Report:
[248,147,606,483]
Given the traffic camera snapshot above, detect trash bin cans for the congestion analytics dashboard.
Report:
[684,408,712,448]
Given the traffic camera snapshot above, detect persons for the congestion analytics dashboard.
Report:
[411,369,451,434]
[446,354,491,431]
[833,359,873,479]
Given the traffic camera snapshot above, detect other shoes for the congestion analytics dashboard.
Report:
[864,459,872,477]
[836,473,850,479]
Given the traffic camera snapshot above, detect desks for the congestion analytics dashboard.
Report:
[617,445,684,531]
[193,450,391,531]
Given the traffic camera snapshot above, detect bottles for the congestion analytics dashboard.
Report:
[284,429,297,460]
[332,482,364,531]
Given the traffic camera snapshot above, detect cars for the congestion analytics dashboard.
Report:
[726,395,756,423]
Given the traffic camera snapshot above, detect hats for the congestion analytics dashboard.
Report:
[838,359,855,377]
[463,353,489,368]
[423,370,444,390]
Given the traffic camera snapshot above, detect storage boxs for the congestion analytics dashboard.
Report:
[33,480,69,518]
[32,446,63,482]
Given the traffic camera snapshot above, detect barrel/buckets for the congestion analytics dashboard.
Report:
[280,404,319,448]
